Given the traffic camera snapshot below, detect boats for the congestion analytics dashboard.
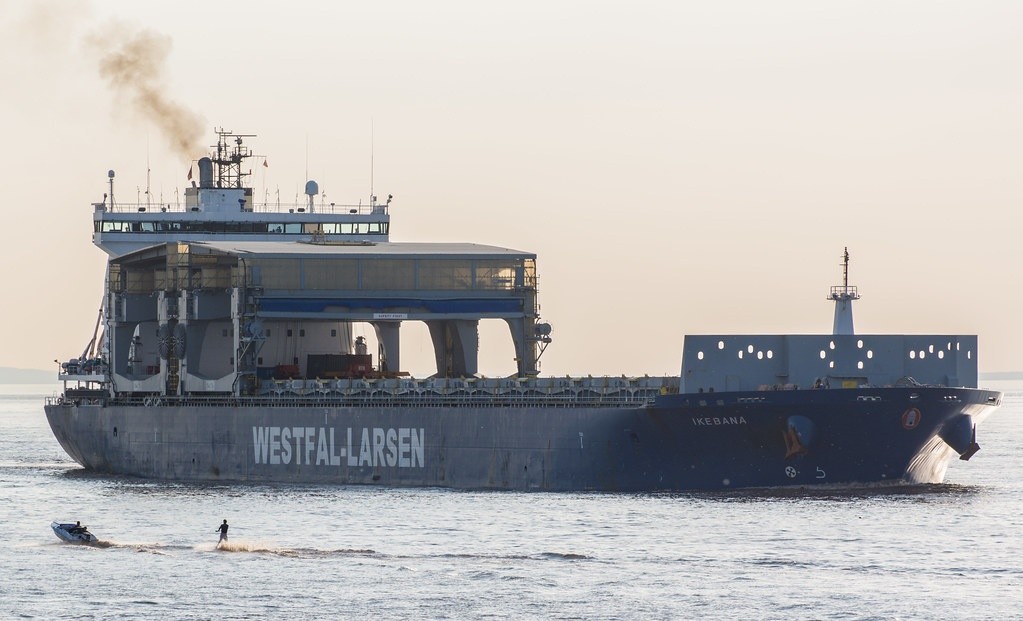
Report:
[44,124,1005,498]
[49,522,101,549]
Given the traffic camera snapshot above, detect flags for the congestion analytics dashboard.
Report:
[188,166,192,180]
[263,160,268,167]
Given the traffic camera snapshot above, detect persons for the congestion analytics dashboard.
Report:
[698,388,703,393]
[822,378,830,389]
[83,527,87,532]
[811,379,821,390]
[278,226,282,232]
[75,521,81,528]
[215,519,229,549]
[708,388,713,392]
